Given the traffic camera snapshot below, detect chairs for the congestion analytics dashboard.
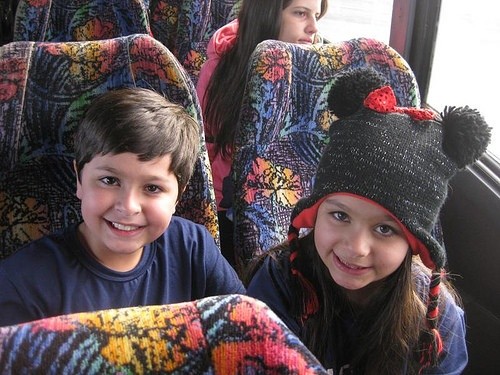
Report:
[0,0,500,375]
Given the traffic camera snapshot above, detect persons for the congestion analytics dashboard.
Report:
[246,66,492,375]
[0,87,247,326]
[196,0,328,221]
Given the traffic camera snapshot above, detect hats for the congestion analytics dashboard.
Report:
[277,69,492,375]
